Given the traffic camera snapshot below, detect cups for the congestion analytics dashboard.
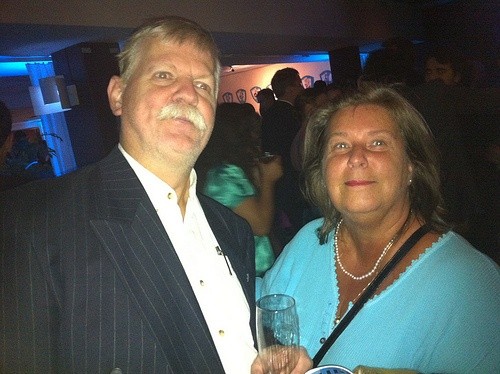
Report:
[255,294,300,374]
[304,365,354,374]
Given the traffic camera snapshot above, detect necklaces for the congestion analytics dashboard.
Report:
[334,205,414,280]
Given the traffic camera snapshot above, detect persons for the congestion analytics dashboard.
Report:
[193,102,285,279]
[0,15,313,374]
[216,41,500,266]
[256,79,500,374]
